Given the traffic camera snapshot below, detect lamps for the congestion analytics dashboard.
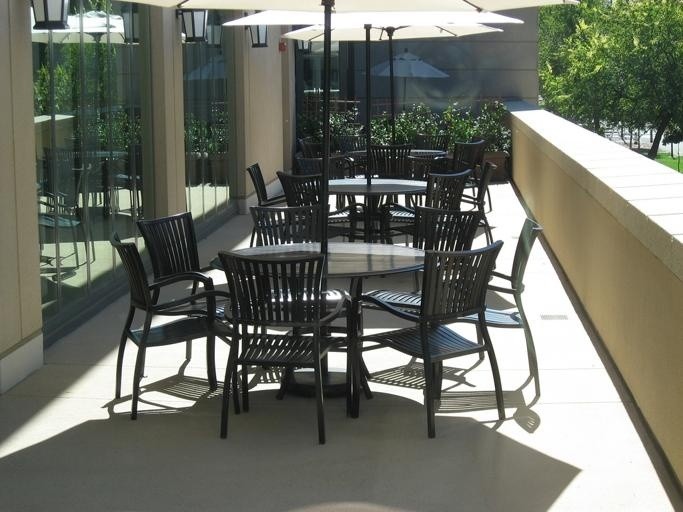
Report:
[39,138,140,269]
[431,139,493,212]
[360,205,485,360]
[217,251,353,444]
[136,210,242,378]
[429,135,451,151]
[333,135,369,176]
[353,239,504,438]
[435,216,544,398]
[371,142,413,207]
[105,231,241,439]
[276,170,367,244]
[248,205,372,379]
[298,137,329,156]
[381,168,473,276]
[427,160,498,248]
[297,156,355,210]
[246,162,290,246]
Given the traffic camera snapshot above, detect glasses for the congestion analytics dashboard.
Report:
[243,9,269,48]
[293,26,313,54]
[30,0,72,30]
[175,10,210,42]
[119,2,142,44]
[204,11,224,48]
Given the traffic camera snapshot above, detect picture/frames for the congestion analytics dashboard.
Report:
[349,149,447,157]
[299,179,437,240]
[209,242,442,399]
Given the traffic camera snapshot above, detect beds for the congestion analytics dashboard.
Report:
[112,0,580,399]
[220,10,525,243]
[31,11,210,215]
[279,23,504,204]
